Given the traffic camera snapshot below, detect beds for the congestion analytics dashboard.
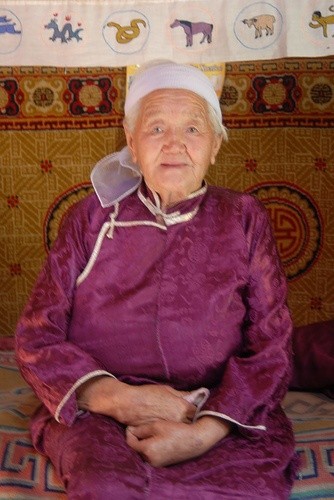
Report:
[0,350,334,500]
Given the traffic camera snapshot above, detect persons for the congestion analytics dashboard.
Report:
[15,63,303,500]
